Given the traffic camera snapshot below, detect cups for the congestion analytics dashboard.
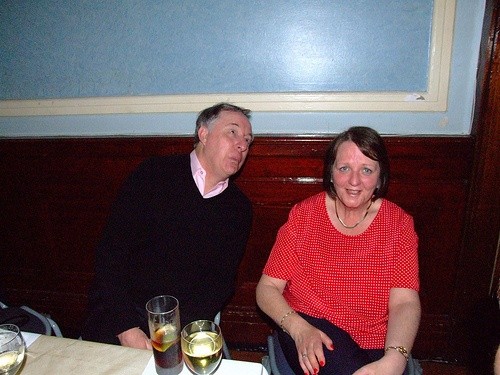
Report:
[181,320,223,375]
[146,295,183,375]
[0,324,25,375]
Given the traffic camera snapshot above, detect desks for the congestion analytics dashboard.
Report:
[15,331,268,375]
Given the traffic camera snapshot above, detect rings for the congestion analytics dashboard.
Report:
[302,354,307,357]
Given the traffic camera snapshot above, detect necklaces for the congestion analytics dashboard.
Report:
[336,196,372,228]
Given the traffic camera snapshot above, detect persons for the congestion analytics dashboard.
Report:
[257,127,421,375]
[86,104,253,344]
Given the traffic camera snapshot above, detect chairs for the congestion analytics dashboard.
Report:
[262,335,423,375]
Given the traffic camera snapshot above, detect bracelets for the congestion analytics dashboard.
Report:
[281,310,296,332]
[385,346,409,361]
